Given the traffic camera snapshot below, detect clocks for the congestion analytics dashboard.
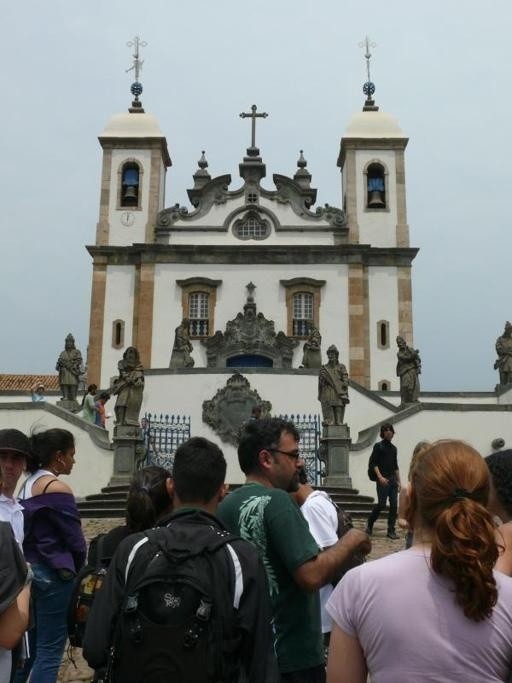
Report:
[120,211,136,227]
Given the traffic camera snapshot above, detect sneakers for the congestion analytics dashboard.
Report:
[367,526,372,533]
[387,533,400,539]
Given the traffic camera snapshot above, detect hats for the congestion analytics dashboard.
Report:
[0,429,31,455]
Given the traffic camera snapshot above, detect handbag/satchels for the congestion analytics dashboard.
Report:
[65,563,111,648]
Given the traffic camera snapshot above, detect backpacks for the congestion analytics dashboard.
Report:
[308,492,366,586]
[116,526,245,683]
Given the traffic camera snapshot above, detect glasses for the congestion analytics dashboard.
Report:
[264,447,300,461]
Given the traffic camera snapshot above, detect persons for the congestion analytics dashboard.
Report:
[141,418,158,465]
[82,437,282,683]
[489,317,512,394]
[57,330,84,404]
[84,384,110,430]
[0,428,86,683]
[396,334,424,404]
[168,313,195,370]
[367,424,510,577]
[327,437,511,683]
[31,383,44,401]
[89,467,174,683]
[111,346,148,428]
[215,420,370,683]
[317,342,352,426]
